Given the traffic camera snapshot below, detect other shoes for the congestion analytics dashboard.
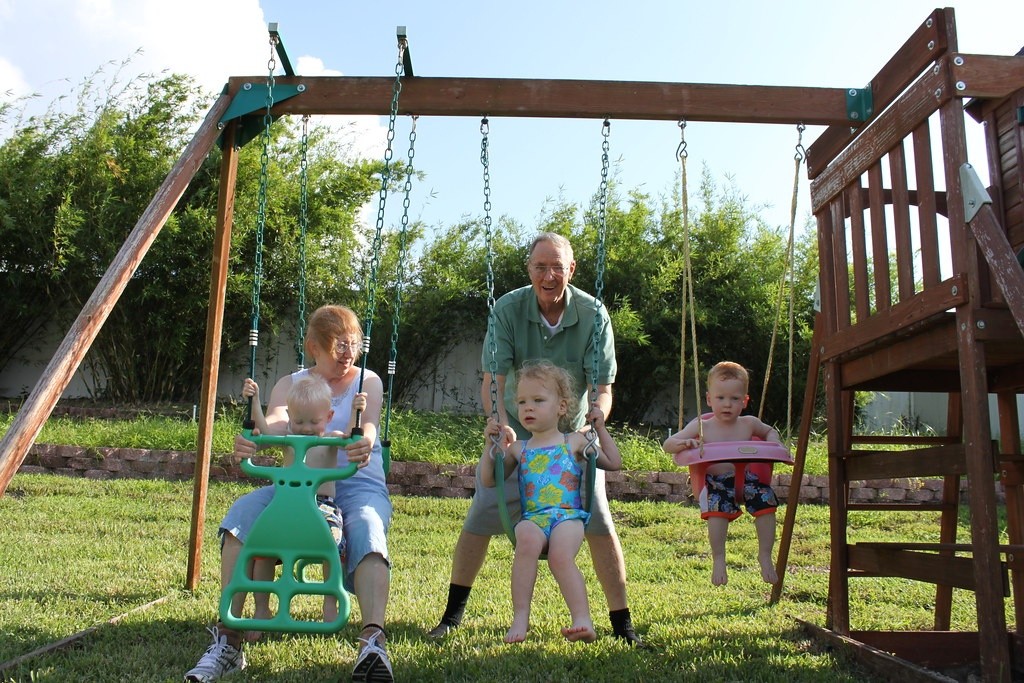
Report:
[612,636,667,656]
[425,623,459,644]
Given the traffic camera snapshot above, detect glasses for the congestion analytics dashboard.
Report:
[334,338,364,353]
[528,258,572,277]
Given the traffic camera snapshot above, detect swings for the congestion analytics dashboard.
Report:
[673,121,806,521]
[472,115,615,562]
[214,37,394,634]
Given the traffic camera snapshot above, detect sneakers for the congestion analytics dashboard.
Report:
[352,630,394,683]
[184,627,246,683]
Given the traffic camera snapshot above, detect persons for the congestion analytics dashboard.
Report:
[662,361,779,587]
[183,306,394,683]
[242,378,368,640]
[479,363,622,644]
[427,233,645,647]
